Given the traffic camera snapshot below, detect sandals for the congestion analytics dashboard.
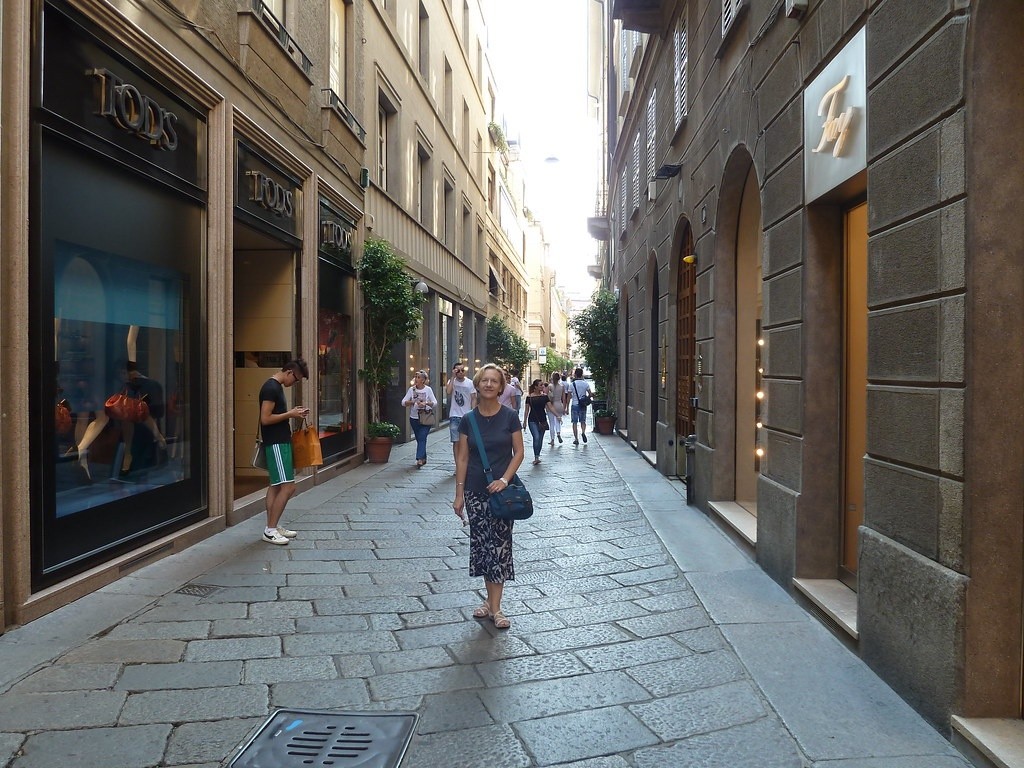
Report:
[473,601,493,618]
[489,609,510,628]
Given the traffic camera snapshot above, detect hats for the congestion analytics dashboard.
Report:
[415,370,428,380]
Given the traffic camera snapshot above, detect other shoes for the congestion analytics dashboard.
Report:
[557,434,562,443]
[118,454,133,481]
[582,433,587,443]
[69,448,89,480]
[533,460,541,465]
[148,437,168,454]
[550,441,554,445]
[417,457,427,468]
[573,440,579,444]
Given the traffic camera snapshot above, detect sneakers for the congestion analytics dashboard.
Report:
[261,527,289,545]
[277,525,298,538]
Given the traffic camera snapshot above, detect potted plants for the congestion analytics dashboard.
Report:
[489,121,509,153]
[593,409,617,436]
[365,421,400,463]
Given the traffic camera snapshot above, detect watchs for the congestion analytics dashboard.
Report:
[500,478,508,486]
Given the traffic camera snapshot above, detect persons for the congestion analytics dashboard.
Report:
[523,379,561,463]
[497,369,524,412]
[452,363,524,628]
[401,370,438,469]
[119,326,169,479]
[560,376,570,403]
[565,367,593,444]
[545,371,566,444]
[447,363,476,461]
[259,358,310,544]
[54,318,110,480]
[543,383,549,394]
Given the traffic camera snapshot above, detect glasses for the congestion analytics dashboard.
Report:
[456,368,465,373]
[288,368,300,382]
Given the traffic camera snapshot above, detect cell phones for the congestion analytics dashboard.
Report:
[304,409,310,412]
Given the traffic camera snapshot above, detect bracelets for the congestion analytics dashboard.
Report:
[456,483,463,485]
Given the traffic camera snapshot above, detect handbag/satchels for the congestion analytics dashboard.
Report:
[546,386,553,402]
[105,393,148,422]
[579,395,591,407]
[55,402,71,434]
[250,442,268,470]
[418,408,436,426]
[489,473,534,520]
[539,421,549,431]
[290,417,323,466]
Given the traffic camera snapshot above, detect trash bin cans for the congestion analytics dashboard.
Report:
[590,399,607,432]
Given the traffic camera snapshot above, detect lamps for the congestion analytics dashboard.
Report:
[413,277,429,294]
[682,255,698,266]
